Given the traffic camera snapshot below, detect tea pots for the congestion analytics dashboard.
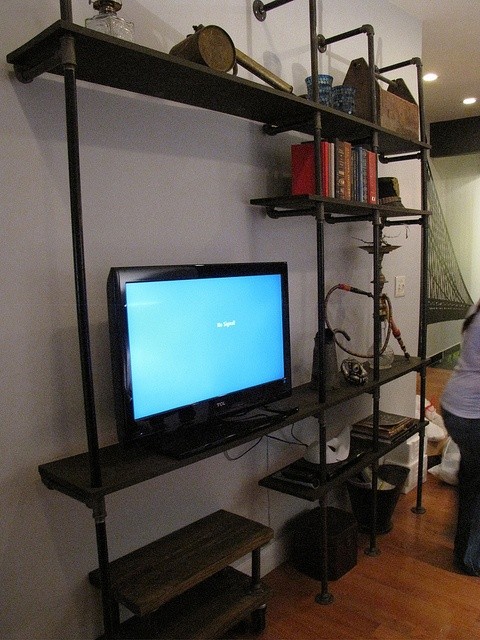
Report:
[310,328,351,393]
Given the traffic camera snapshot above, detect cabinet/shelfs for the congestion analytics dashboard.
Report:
[6,0,460,640]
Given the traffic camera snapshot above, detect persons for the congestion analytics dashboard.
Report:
[444,301,479,580]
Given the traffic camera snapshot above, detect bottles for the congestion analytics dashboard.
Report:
[367,322,394,370]
[85,0,135,43]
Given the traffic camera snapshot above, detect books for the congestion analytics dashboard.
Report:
[350,412,418,448]
[291,139,382,203]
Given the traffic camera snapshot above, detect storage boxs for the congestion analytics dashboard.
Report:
[342,58,421,143]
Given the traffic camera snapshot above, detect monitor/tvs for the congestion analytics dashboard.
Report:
[107,261,293,458]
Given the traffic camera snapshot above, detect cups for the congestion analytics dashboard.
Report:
[332,85,358,116]
[304,74,334,108]
